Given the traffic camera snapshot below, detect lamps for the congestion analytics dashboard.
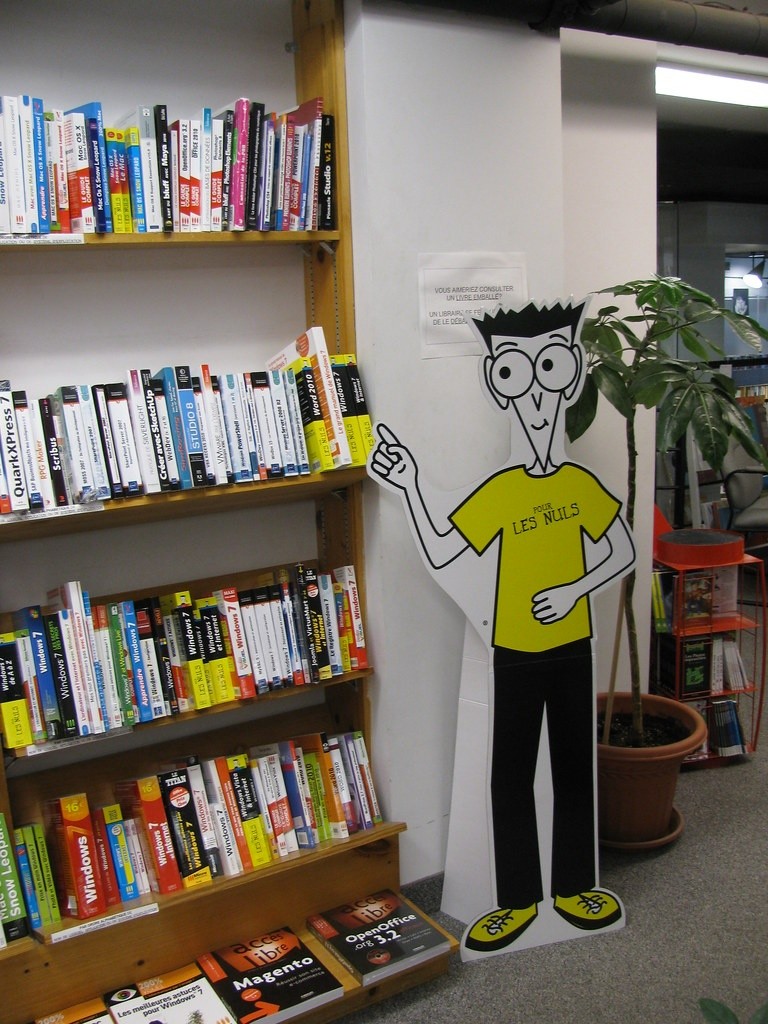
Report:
[743,262,764,288]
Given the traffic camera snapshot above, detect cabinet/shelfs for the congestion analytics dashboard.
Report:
[1,0,458,1024]
[648,504,767,764]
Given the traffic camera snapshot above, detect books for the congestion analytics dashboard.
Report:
[0,730,386,945]
[650,561,751,763]
[1,324,377,516]
[32,997,117,1024]
[104,961,239,1024]
[0,562,369,758]
[194,926,344,1024]
[0,93,338,238]
[305,888,451,987]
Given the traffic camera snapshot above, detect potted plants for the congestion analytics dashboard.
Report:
[565,275,768,855]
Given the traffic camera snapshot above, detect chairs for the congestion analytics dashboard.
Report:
[720,433,768,537]
